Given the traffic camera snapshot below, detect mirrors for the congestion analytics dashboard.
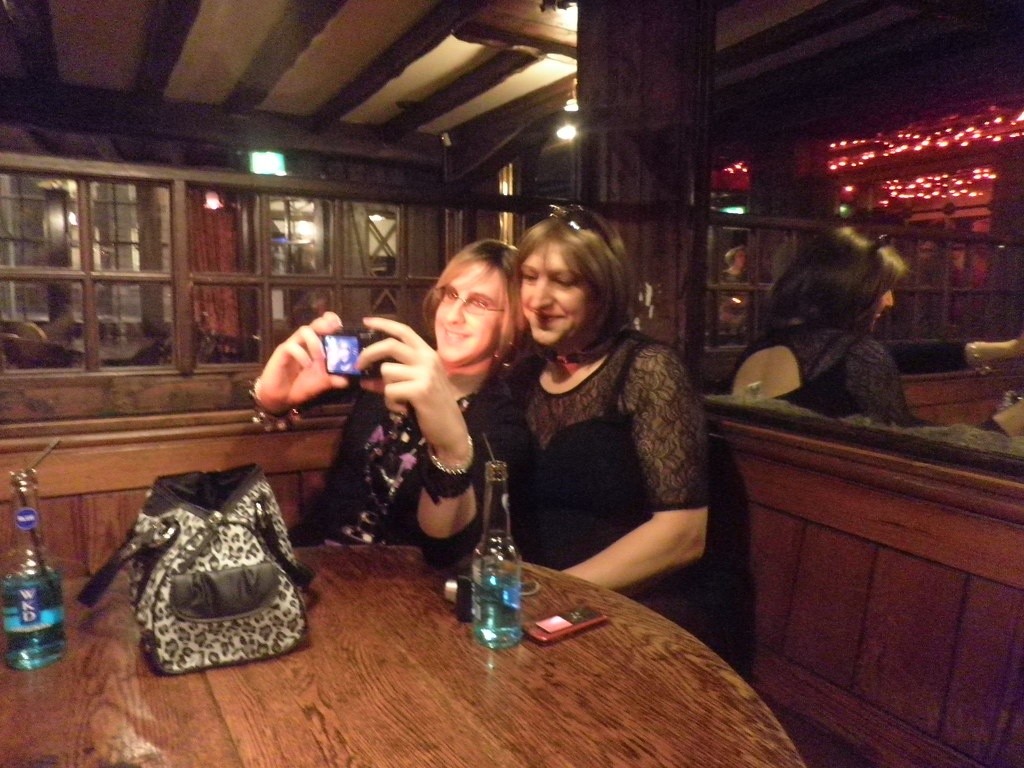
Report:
[680,0,1024,479]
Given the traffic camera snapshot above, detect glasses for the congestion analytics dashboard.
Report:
[873,231,891,247]
[440,285,505,315]
[549,200,616,253]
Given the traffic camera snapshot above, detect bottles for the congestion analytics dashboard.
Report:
[472,461,522,649]
[0,469,66,671]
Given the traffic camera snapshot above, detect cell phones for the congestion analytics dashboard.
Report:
[518,603,609,646]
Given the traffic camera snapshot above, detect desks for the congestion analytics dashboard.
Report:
[72,335,158,371]
[0,547,806,768]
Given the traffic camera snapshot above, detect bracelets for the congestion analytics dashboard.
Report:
[247,379,304,434]
[425,435,476,477]
[968,341,992,373]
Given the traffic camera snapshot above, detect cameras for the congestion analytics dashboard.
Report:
[322,325,386,379]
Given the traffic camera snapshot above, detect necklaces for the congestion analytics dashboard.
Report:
[544,351,590,382]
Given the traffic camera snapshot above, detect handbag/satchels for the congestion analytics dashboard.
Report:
[78,461,315,678]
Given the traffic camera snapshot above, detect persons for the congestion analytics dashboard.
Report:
[718,223,1024,442]
[509,202,713,692]
[242,236,536,582]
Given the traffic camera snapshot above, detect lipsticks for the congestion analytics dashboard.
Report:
[454,574,473,623]
